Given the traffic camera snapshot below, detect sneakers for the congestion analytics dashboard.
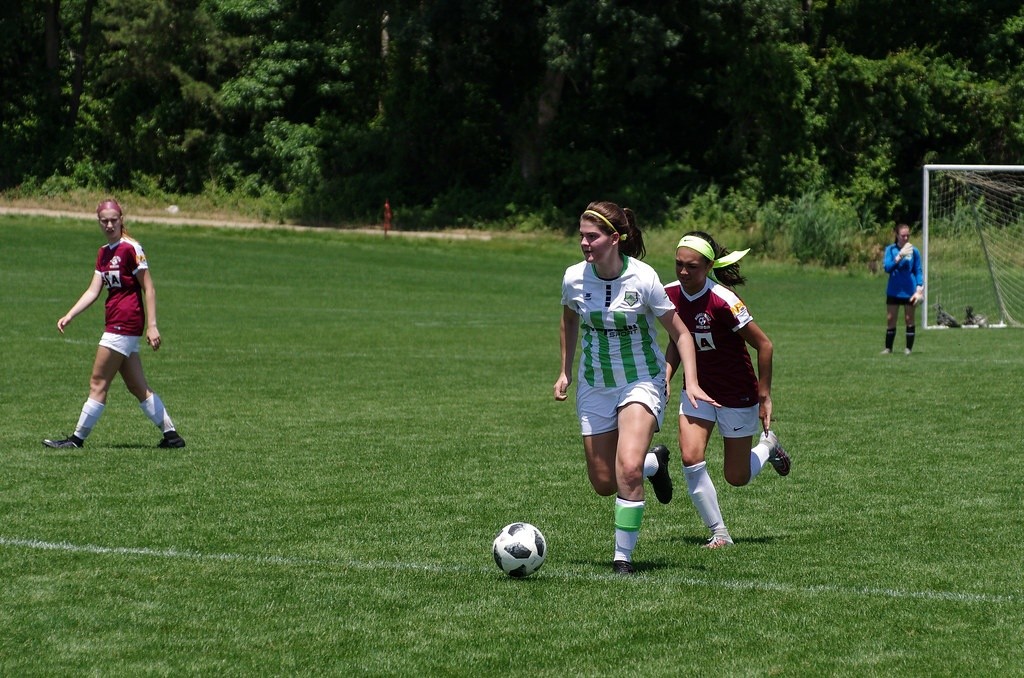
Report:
[156,437,185,448]
[760,430,791,476]
[701,533,734,550]
[42,432,83,450]
[647,445,673,504]
[613,560,634,577]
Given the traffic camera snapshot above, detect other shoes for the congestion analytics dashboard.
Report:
[881,349,894,355]
[905,348,911,356]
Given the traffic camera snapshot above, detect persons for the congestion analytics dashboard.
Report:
[554,201,721,575]
[662,232,791,546]
[41,199,186,449]
[880,224,924,354]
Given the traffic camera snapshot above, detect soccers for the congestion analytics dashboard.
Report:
[491,521,548,578]
